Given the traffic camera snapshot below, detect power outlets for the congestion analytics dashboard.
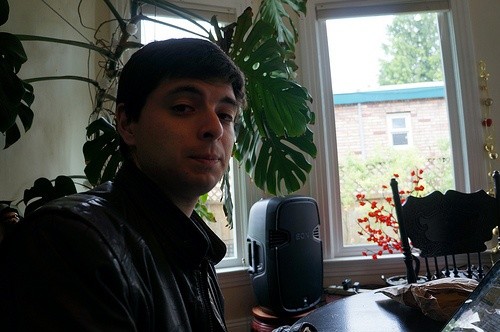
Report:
[328,284,373,296]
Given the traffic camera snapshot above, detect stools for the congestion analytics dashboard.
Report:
[251,293,345,332]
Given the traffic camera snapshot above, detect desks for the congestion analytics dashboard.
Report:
[288,282,453,332]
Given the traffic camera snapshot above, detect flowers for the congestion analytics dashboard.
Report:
[356,169,424,281]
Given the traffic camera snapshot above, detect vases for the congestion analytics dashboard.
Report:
[386,275,428,287]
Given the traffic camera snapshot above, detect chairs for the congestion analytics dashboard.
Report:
[390,170,500,284]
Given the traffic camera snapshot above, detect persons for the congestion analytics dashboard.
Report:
[0,37,250,332]
